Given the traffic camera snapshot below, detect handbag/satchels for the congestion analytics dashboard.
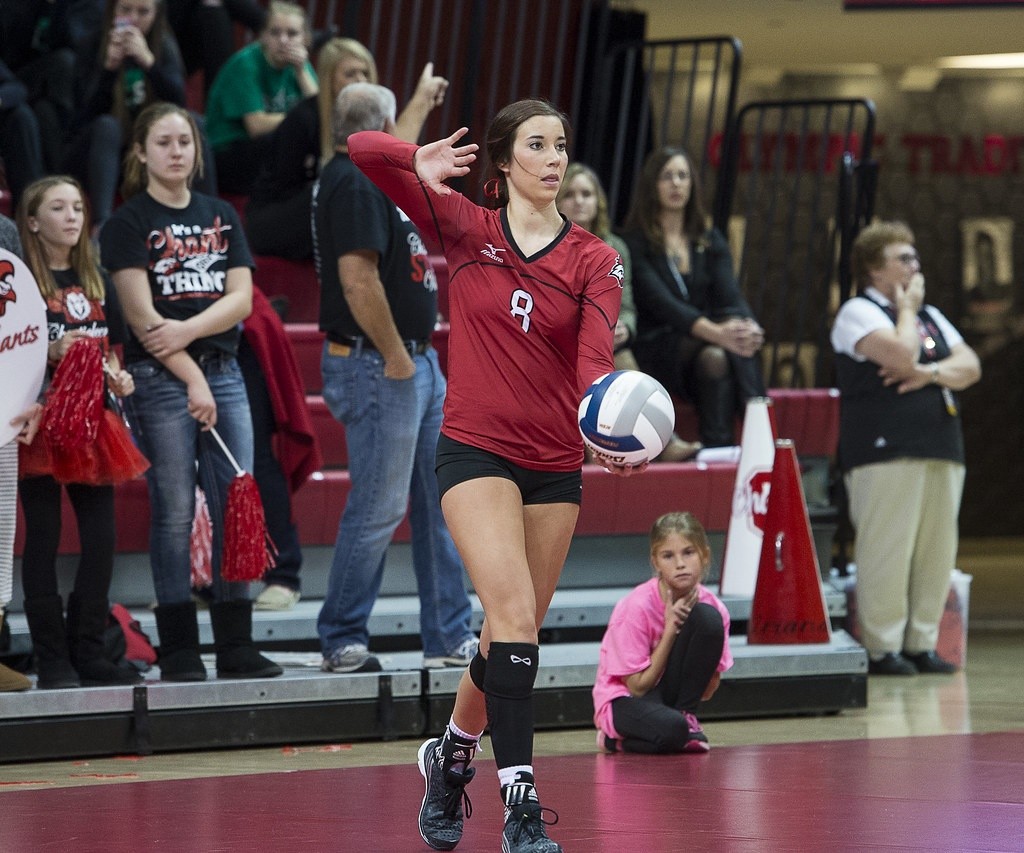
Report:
[935,566,973,672]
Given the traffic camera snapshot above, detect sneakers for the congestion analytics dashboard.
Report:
[501,803,565,853]
[596,729,621,753]
[321,642,383,673]
[417,738,477,852]
[678,706,710,753]
[422,636,481,668]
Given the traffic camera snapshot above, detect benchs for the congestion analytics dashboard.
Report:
[0,183,737,557]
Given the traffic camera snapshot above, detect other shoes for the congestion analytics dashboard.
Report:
[868,651,918,675]
[0,663,32,692]
[659,434,703,463]
[252,584,302,611]
[901,647,956,673]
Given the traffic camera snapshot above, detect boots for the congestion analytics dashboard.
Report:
[65,590,142,684]
[24,593,81,688]
[210,596,284,678]
[154,600,206,682]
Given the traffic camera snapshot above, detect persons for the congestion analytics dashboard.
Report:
[0,0,380,263]
[314,63,484,671]
[11,177,131,688]
[592,512,732,754]
[98,104,283,681]
[837,220,980,677]
[347,98,650,844]
[0,213,48,694]
[557,163,702,464]
[619,140,772,447]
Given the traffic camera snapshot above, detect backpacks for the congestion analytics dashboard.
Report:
[102,602,156,673]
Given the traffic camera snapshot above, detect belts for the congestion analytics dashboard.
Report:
[325,328,433,358]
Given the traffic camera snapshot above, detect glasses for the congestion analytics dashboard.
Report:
[899,253,920,263]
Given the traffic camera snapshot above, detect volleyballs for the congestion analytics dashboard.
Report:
[577,370,676,468]
